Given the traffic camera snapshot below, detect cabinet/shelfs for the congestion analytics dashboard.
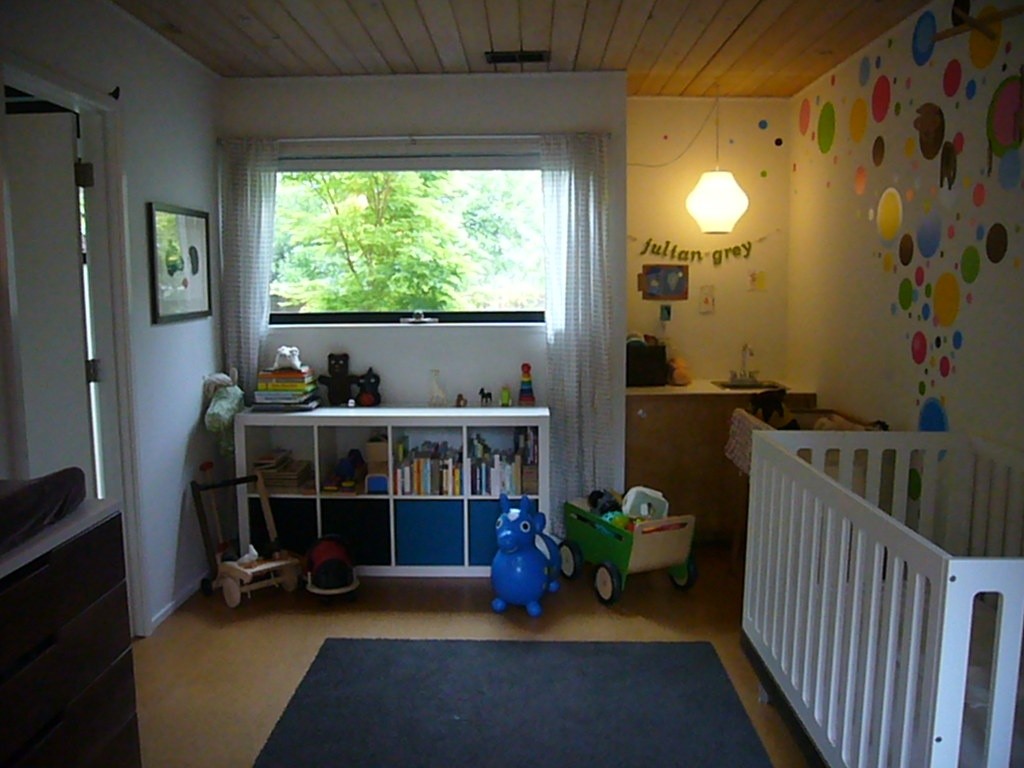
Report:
[229,395,553,582]
[2,469,142,767]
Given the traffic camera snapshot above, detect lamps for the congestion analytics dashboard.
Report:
[684,84,748,232]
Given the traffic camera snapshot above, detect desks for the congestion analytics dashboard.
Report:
[627,381,783,543]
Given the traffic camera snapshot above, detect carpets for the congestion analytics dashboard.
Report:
[251,636,773,768]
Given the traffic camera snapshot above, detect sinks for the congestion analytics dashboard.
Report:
[710,377,791,393]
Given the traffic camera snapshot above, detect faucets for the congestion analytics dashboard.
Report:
[738,342,756,383]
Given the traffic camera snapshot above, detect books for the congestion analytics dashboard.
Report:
[245,363,325,413]
[249,428,540,498]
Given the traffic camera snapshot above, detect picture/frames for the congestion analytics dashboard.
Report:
[145,197,213,324]
